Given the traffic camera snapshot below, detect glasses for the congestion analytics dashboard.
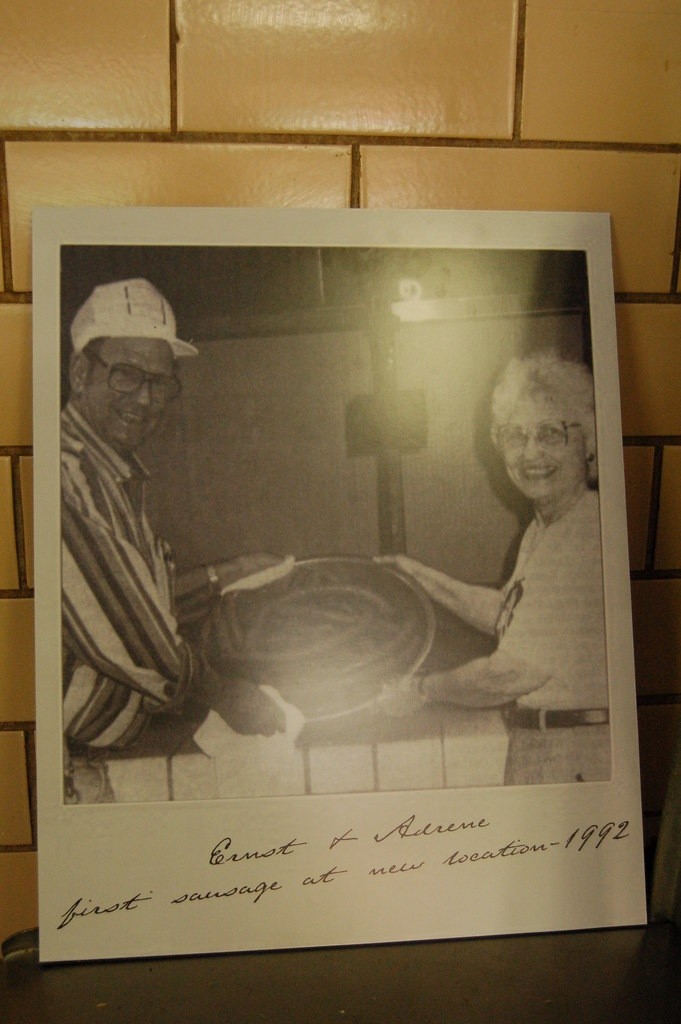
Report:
[496,419,583,447]
[83,348,181,404]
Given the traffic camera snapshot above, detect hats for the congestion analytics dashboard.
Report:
[70,277,199,360]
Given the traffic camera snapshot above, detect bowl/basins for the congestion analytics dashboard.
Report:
[199,555,438,729]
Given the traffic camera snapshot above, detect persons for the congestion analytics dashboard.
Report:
[60,278,287,805]
[372,356,613,784]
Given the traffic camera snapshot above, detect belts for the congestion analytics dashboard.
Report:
[501,707,610,729]
[65,736,211,762]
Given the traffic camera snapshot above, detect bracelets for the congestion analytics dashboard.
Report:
[207,564,219,590]
[418,671,431,704]
